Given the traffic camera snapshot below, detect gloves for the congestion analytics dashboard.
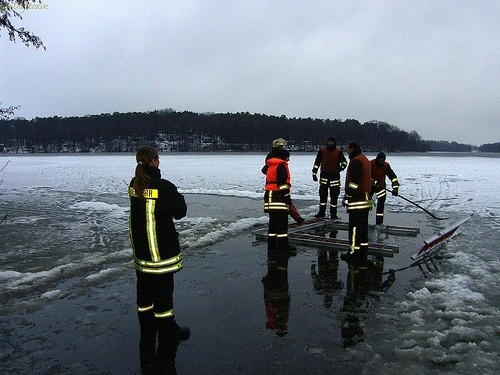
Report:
[282,190,292,204]
[342,195,352,206]
[376,183,383,191]
[336,166,342,173]
[313,175,318,181]
[392,188,398,196]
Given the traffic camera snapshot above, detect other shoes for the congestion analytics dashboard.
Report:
[297,217,306,225]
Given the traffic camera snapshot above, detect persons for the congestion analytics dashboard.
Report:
[312,137,347,219]
[368,256,396,302]
[339,264,367,349]
[311,228,345,309]
[369,152,399,231]
[340,142,372,262]
[128,146,190,338]
[261,137,305,252]
[265,250,297,337]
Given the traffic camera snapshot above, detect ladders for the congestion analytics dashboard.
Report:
[328,222,421,237]
[411,214,473,262]
[251,216,341,244]
[287,233,399,256]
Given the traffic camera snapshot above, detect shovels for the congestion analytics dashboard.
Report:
[386,187,450,220]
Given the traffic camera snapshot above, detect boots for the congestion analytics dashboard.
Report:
[138,309,158,347]
[278,237,297,252]
[359,245,368,260]
[267,236,278,250]
[314,205,326,218]
[155,316,191,344]
[330,206,339,219]
[340,248,359,261]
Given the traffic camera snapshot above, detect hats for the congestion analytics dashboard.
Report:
[278,149,290,160]
[271,138,285,148]
[376,152,386,166]
